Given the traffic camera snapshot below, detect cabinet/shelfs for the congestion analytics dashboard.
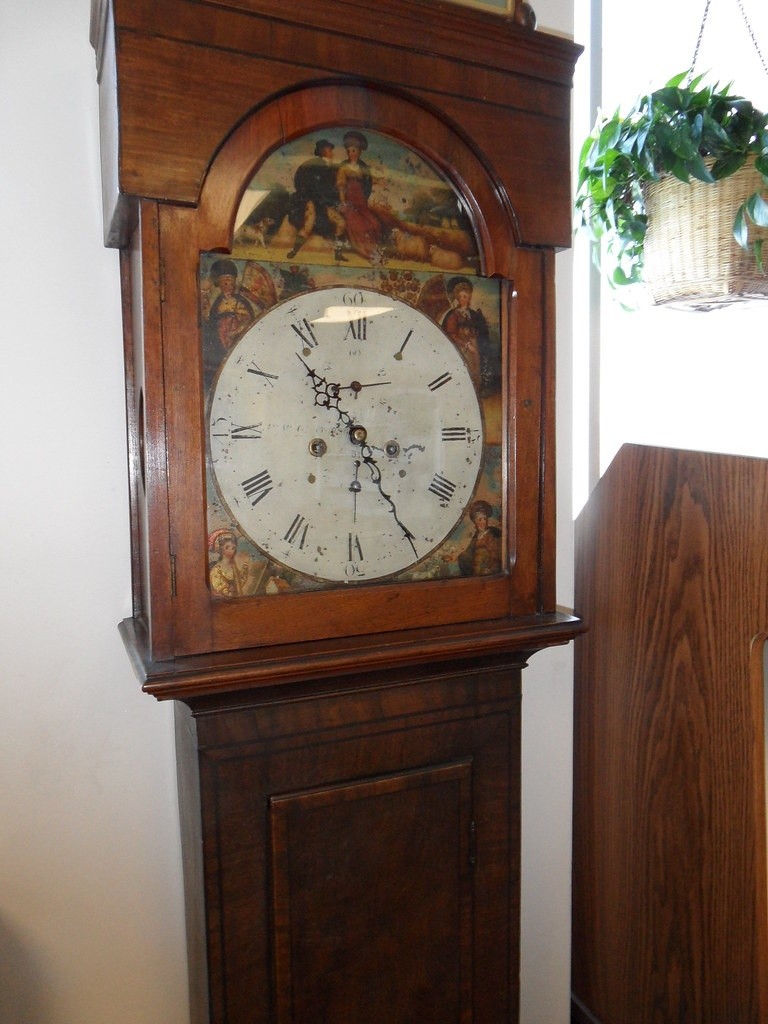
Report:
[114,603,585,1024]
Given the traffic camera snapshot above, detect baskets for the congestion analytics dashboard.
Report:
[638,150,768,311]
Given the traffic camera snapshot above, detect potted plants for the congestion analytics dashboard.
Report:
[569,66,768,313]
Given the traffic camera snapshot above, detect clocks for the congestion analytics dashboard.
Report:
[91,0,589,663]
